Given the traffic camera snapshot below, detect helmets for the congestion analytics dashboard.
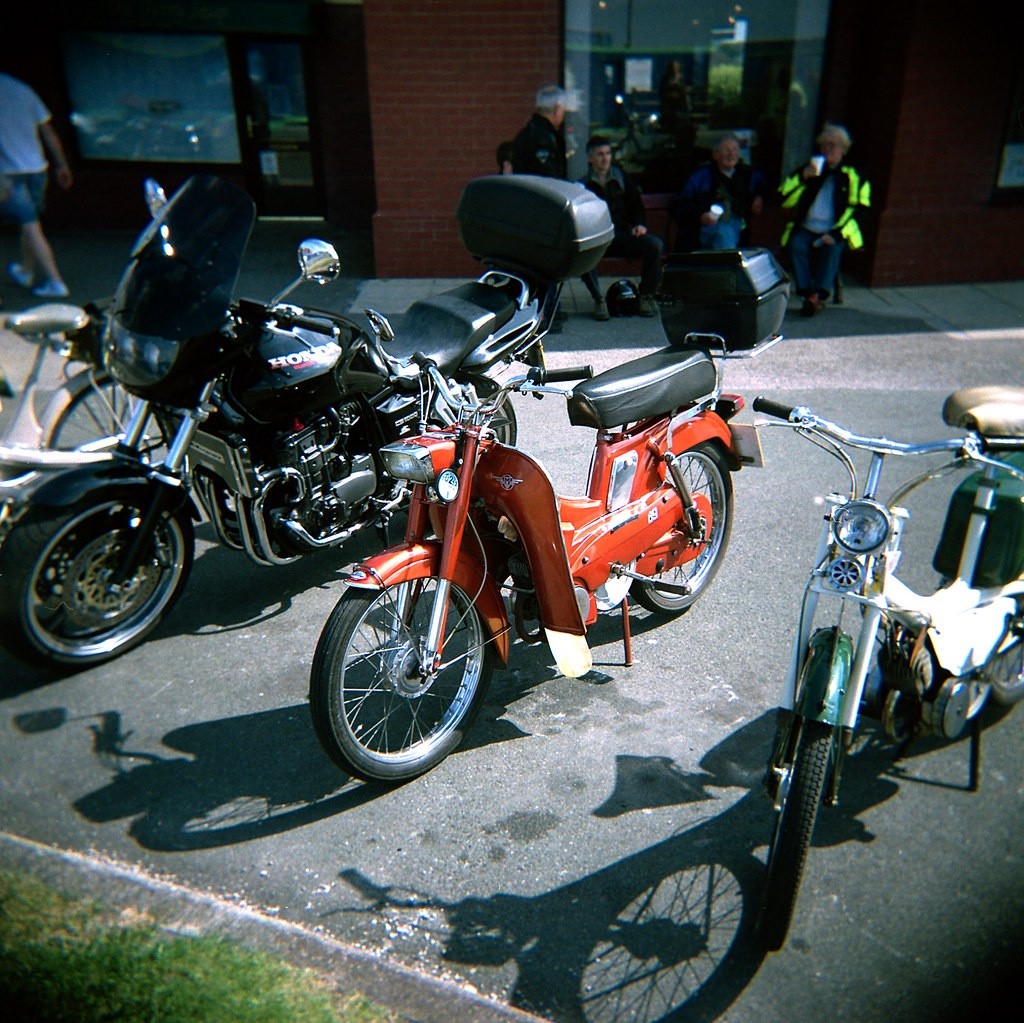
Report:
[605,279,639,317]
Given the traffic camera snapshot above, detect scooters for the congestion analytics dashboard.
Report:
[309,301,765,786]
[743,384,1023,952]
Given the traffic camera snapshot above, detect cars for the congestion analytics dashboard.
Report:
[219,104,323,191]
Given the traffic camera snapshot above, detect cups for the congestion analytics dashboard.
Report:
[810,156,825,176]
[709,204,724,221]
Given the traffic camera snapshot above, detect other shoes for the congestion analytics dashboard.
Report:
[801,293,824,316]
[638,288,657,316]
[548,311,569,321]
[594,298,609,320]
[538,314,562,334]
[33,281,70,297]
[11,264,34,286]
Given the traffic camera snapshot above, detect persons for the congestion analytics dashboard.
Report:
[773,123,872,317]
[576,137,663,320]
[496,86,568,332]
[679,138,771,250]
[0,74,74,297]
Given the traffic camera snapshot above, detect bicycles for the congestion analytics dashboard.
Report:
[0,304,186,542]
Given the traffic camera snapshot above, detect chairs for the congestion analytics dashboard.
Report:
[669,162,844,304]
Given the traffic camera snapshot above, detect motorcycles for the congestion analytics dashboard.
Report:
[0,162,619,669]
